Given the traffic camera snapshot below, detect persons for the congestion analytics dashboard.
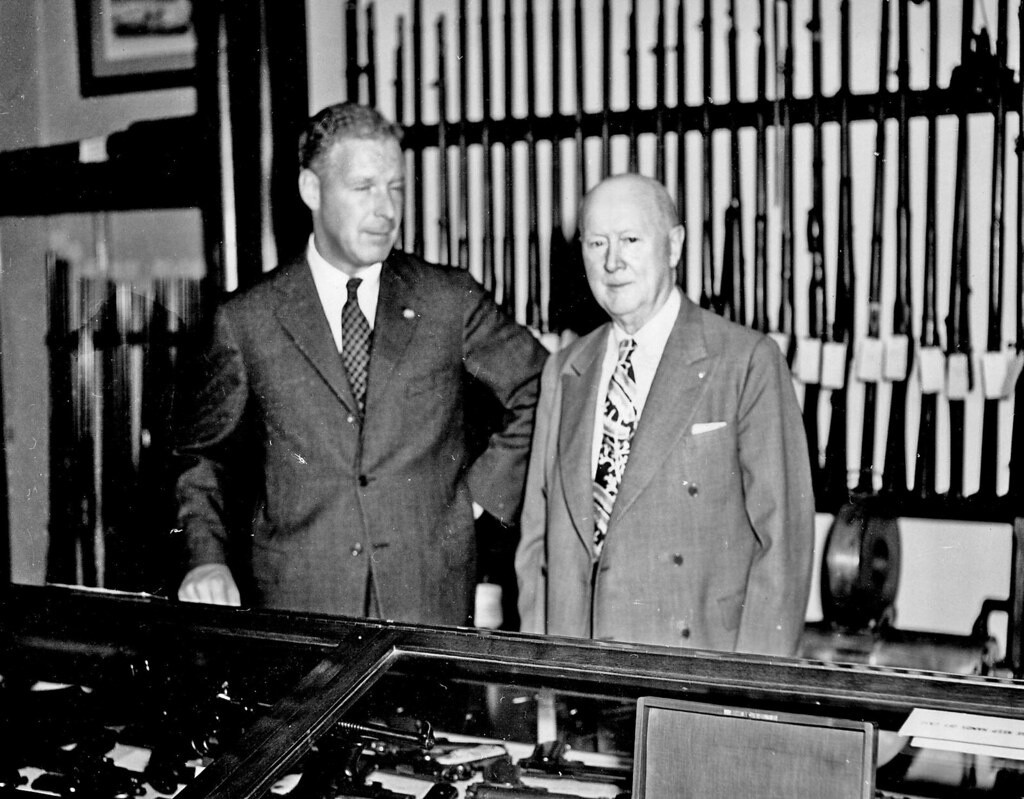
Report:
[177,102,550,630]
[515,174,814,661]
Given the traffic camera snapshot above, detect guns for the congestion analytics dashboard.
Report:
[0,658,633,799]
[333,1,1024,513]
[41,262,204,595]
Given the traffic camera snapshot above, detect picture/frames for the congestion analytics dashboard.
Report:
[73,1,199,100]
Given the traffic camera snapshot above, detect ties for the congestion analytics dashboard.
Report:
[590,339,639,557]
[340,278,373,418]
